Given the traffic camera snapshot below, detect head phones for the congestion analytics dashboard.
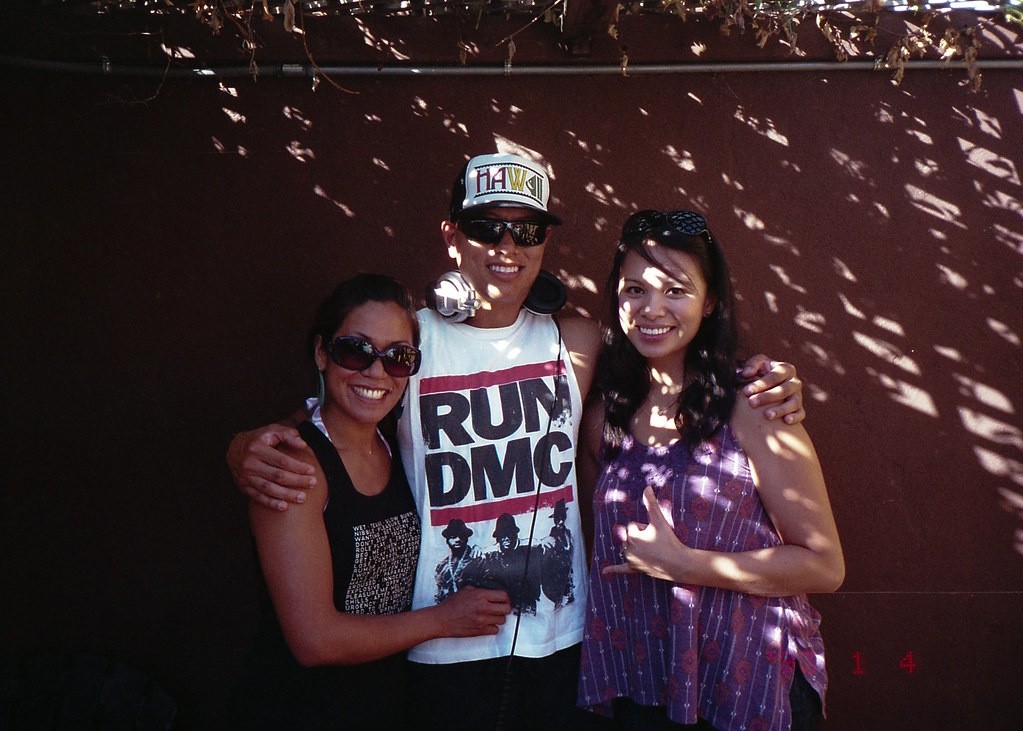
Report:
[425,269,568,323]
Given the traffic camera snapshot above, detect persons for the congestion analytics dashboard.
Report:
[576,210,845,731]
[224,151,805,731]
[243,276,511,731]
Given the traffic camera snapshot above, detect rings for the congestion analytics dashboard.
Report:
[619,548,627,560]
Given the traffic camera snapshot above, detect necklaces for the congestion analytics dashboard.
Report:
[645,391,680,415]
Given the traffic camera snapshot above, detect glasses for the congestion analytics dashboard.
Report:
[324,335,422,378]
[621,209,712,244]
[454,217,548,247]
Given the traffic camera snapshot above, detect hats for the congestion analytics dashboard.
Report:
[449,153,564,228]
